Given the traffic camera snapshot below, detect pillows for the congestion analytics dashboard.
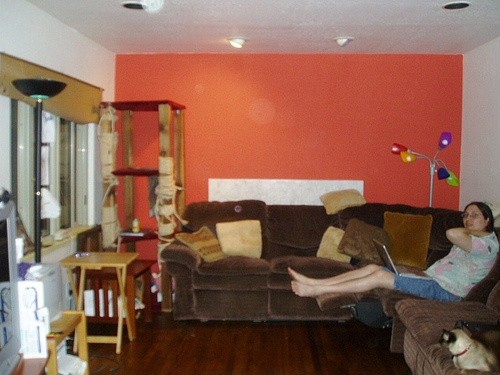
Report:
[336,218,390,265]
[316,226,352,263]
[216,220,263,259]
[319,188,367,215]
[175,225,229,263]
[383,211,433,269]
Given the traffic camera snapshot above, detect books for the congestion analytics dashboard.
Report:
[119,226,153,237]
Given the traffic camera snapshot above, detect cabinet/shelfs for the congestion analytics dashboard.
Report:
[12,350,52,375]
[44,311,91,375]
[97,98,186,312]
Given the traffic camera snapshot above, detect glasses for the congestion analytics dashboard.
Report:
[460,211,482,219]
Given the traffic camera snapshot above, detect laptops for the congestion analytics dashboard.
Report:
[372,238,437,282]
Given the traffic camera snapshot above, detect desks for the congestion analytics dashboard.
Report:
[72,259,158,338]
[59,251,140,354]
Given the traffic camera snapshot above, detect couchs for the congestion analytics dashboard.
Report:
[162,198,500,375]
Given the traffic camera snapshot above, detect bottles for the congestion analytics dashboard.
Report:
[131,217,141,233]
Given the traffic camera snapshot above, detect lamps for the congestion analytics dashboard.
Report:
[11,79,66,264]
[391,132,463,208]
[230,39,245,49]
[336,38,349,47]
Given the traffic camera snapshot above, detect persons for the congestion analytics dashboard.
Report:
[288,202,499,304]
